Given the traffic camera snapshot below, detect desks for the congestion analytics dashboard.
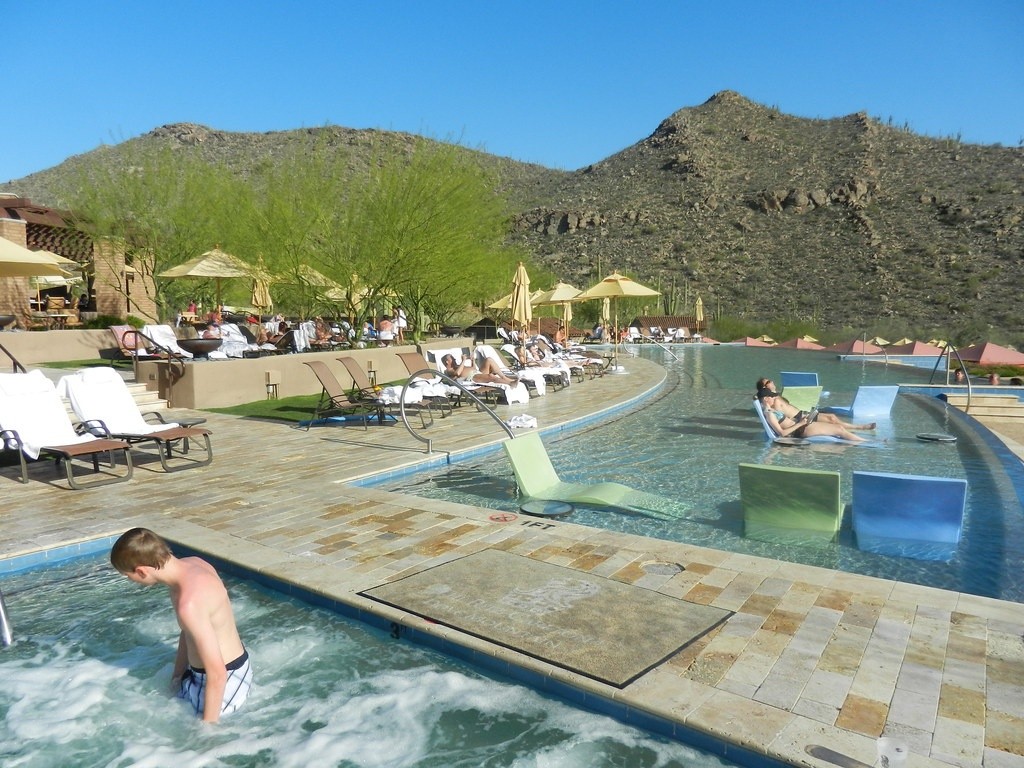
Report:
[520,500,574,517]
[47,314,74,330]
[916,433,958,442]
[773,438,812,445]
[164,418,206,454]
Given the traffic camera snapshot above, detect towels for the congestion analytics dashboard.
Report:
[263,320,311,351]
[55,367,180,436]
[130,349,154,357]
[668,327,686,341]
[141,325,193,356]
[625,326,641,343]
[373,375,462,405]
[377,330,393,340]
[192,324,278,358]
[393,310,405,336]
[0,367,103,460]
[650,325,673,344]
[424,327,602,405]
[300,320,349,347]
[506,412,538,429]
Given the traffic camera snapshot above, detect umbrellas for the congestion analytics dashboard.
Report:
[0,234,78,331]
[488,260,663,371]
[694,296,704,342]
[157,242,404,348]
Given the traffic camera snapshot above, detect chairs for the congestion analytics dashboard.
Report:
[738,463,845,550]
[427,327,702,402]
[335,357,455,418]
[852,471,967,562]
[58,309,82,329]
[502,432,691,521]
[108,308,397,367]
[56,367,213,473]
[780,372,830,398]
[781,386,823,412]
[21,308,50,331]
[0,369,133,489]
[753,392,881,448]
[818,386,899,418]
[395,353,497,412]
[301,361,435,432]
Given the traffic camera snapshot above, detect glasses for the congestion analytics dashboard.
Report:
[765,380,770,386]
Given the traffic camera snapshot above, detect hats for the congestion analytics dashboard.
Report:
[757,388,778,398]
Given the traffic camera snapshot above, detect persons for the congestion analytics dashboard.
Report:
[391,306,399,346]
[441,352,519,388]
[553,325,567,344]
[988,373,1001,385]
[252,320,288,346]
[188,298,198,321]
[397,304,408,345]
[954,369,965,381]
[110,527,254,722]
[756,378,889,444]
[379,314,395,347]
[514,344,591,366]
[1009,378,1022,386]
[315,319,347,342]
